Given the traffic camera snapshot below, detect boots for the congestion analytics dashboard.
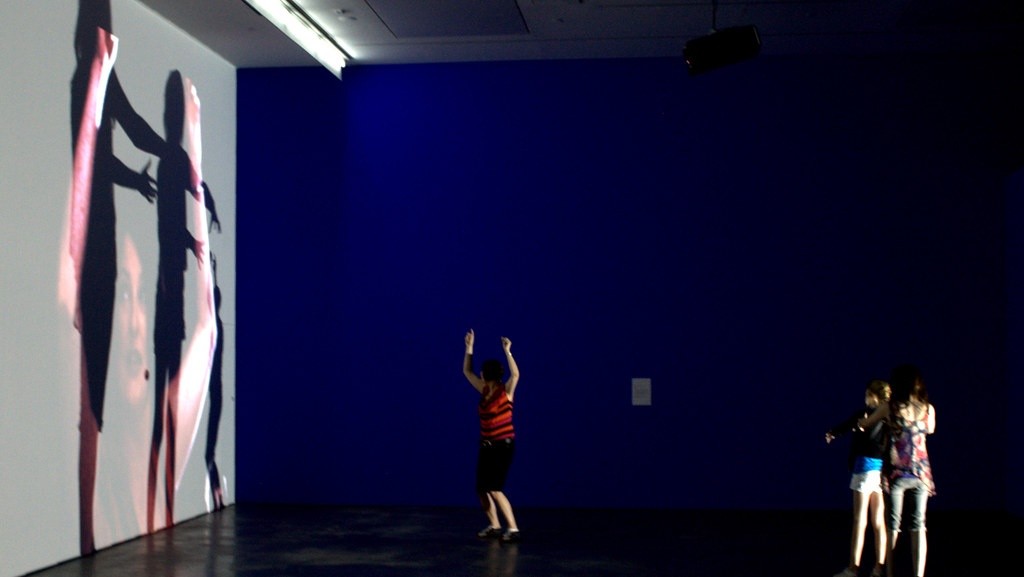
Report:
[870,562,884,577]
[832,564,859,577]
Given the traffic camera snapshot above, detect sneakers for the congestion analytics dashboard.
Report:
[478,526,502,537]
[503,529,520,540]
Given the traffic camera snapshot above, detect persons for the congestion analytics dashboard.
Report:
[462,329,521,542]
[881,363,936,577]
[826,379,893,577]
